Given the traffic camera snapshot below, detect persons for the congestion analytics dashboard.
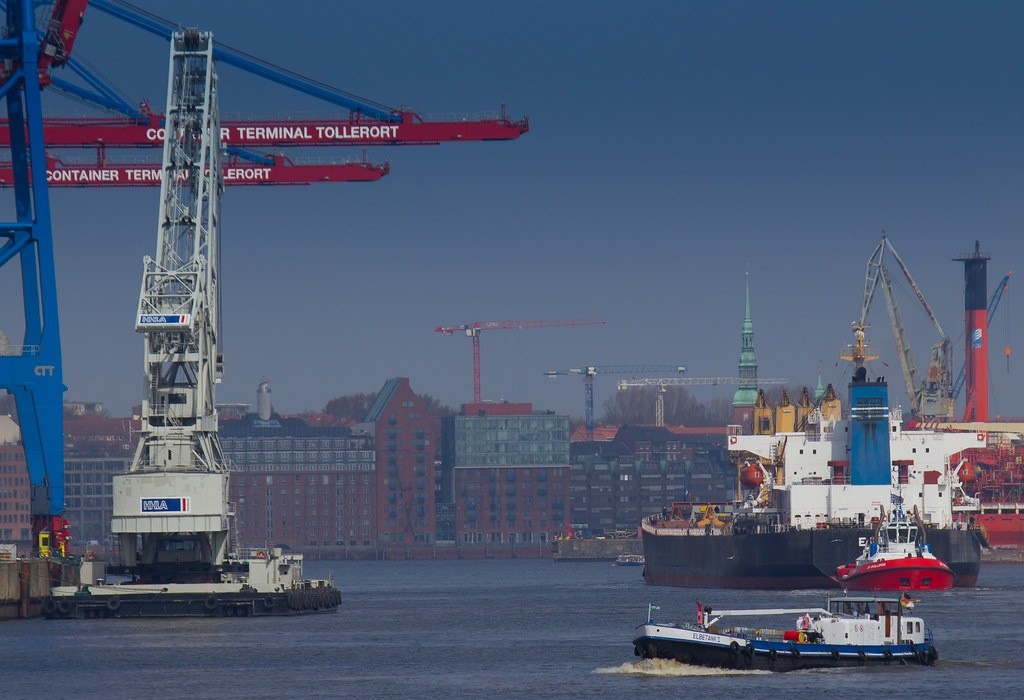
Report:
[798,615,823,644]
[850,602,892,621]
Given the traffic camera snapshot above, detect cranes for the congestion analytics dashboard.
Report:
[433,317,607,418]
[540,364,688,455]
[616,376,788,430]
[851,229,957,424]
[0,1,541,616]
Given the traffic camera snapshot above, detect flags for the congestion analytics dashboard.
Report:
[900,592,921,607]
[649,603,661,613]
[890,494,905,506]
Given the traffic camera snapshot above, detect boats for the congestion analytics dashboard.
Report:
[632,588,935,675]
[835,482,957,592]
[615,553,646,566]
